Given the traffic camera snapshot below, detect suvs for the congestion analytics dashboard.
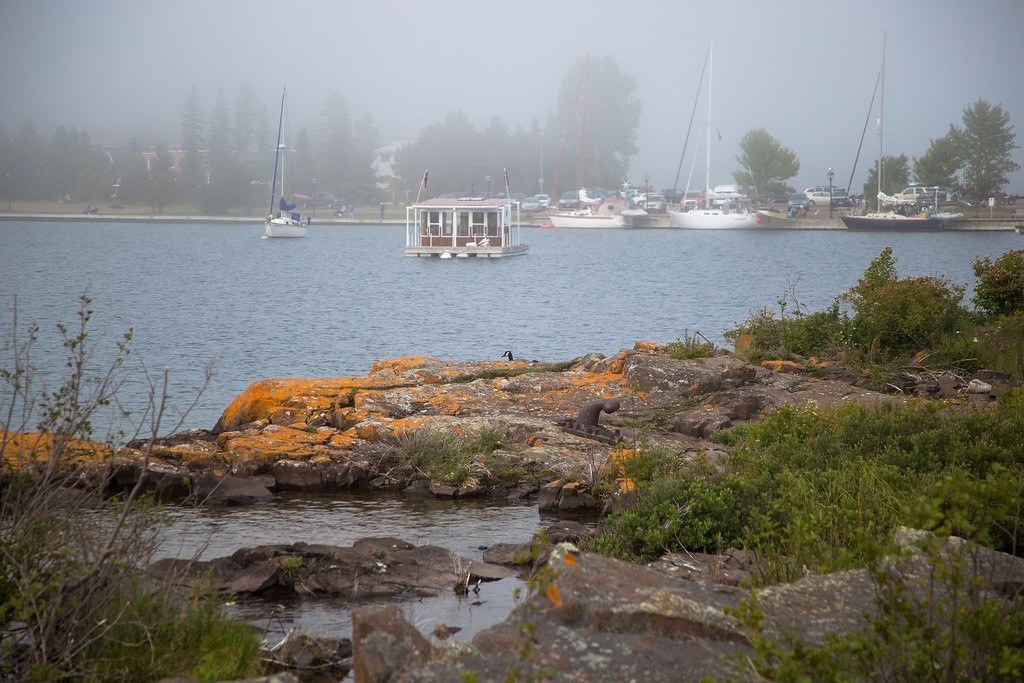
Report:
[893,186,934,201]
[680,190,713,210]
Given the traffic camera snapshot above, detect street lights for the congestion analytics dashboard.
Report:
[643,171,650,212]
[828,167,834,218]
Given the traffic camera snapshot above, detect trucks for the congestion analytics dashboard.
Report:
[714,184,745,210]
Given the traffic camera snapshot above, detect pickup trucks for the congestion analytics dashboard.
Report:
[534,194,552,209]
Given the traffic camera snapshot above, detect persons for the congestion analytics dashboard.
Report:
[380,201,385,220]
[850,194,867,216]
[797,204,810,228]
[348,202,355,219]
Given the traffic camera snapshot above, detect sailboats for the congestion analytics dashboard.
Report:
[546,69,650,229]
[840,28,947,232]
[664,37,761,230]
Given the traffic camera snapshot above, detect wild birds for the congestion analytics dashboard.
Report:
[502,351,540,362]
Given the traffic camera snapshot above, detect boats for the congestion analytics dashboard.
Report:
[405,168,530,257]
[263,85,310,238]
[754,204,851,233]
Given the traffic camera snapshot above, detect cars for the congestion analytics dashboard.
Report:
[762,183,849,213]
[581,182,683,213]
[438,189,525,203]
[558,190,581,210]
[957,198,978,209]
[302,192,344,209]
[521,197,543,212]
